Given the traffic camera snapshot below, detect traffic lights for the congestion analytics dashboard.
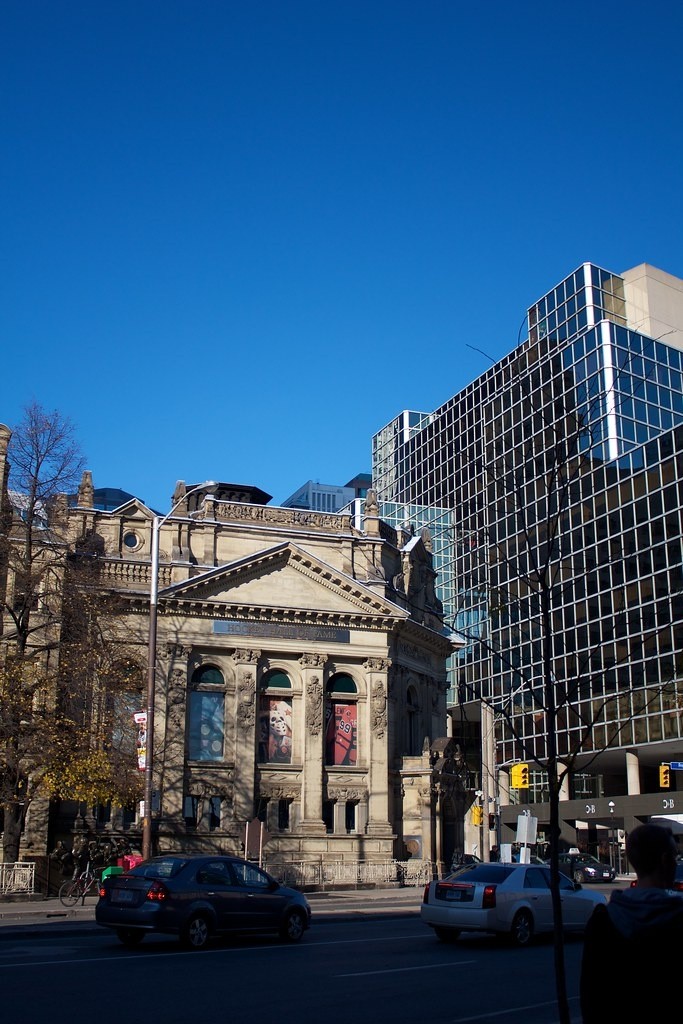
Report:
[658,765,670,789]
[517,764,529,791]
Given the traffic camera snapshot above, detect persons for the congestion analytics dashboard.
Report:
[449,839,590,877]
[45,833,133,887]
[579,823,683,1024]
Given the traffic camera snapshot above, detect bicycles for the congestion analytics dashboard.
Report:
[58,866,104,907]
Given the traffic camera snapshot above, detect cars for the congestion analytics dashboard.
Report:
[418,862,607,948]
[93,853,312,954]
[448,847,616,886]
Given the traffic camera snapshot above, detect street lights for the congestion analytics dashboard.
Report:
[476,668,554,862]
[139,480,219,861]
